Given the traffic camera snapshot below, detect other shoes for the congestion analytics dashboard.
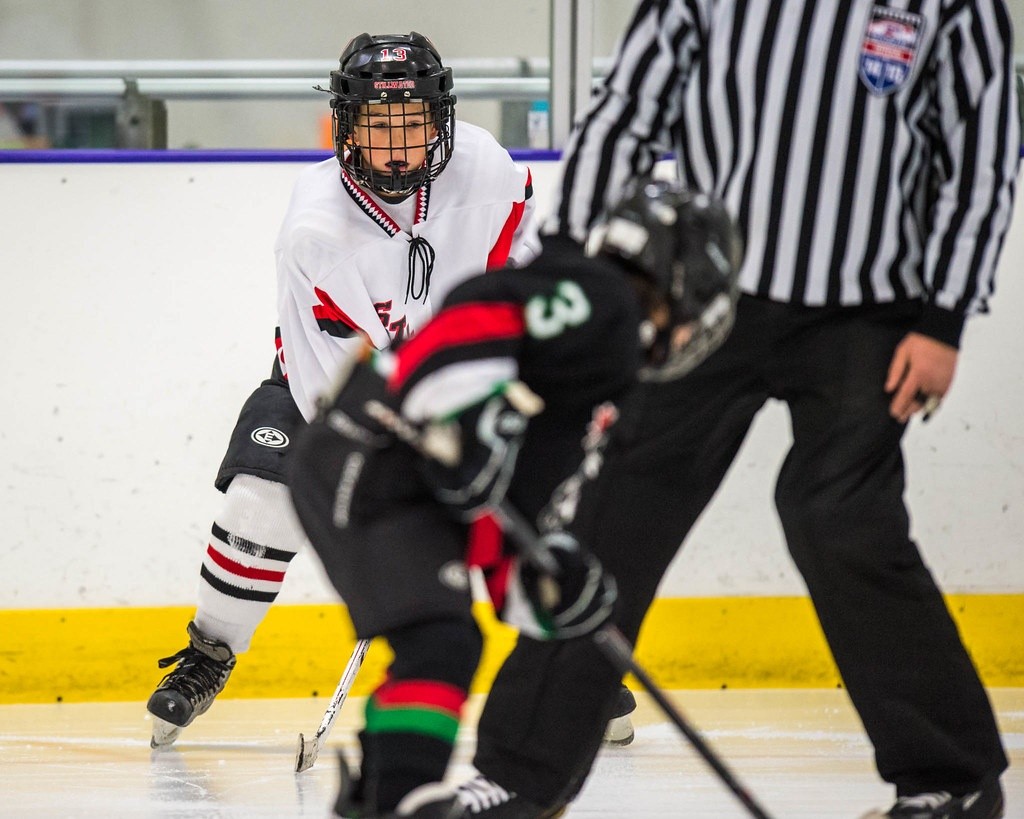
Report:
[334,728,456,819]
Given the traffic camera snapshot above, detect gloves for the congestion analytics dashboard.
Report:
[515,535,616,643]
[413,396,531,524]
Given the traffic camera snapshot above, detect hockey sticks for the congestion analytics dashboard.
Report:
[358,396,777,817]
[292,634,373,775]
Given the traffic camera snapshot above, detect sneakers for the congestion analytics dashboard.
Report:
[883,779,1004,819]
[145,617,237,760]
[394,777,566,819]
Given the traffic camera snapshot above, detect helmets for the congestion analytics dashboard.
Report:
[581,177,749,379]
[329,31,457,200]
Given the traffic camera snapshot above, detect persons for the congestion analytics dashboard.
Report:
[141,30,534,751]
[395,0,1024,818]
[289,175,742,818]
